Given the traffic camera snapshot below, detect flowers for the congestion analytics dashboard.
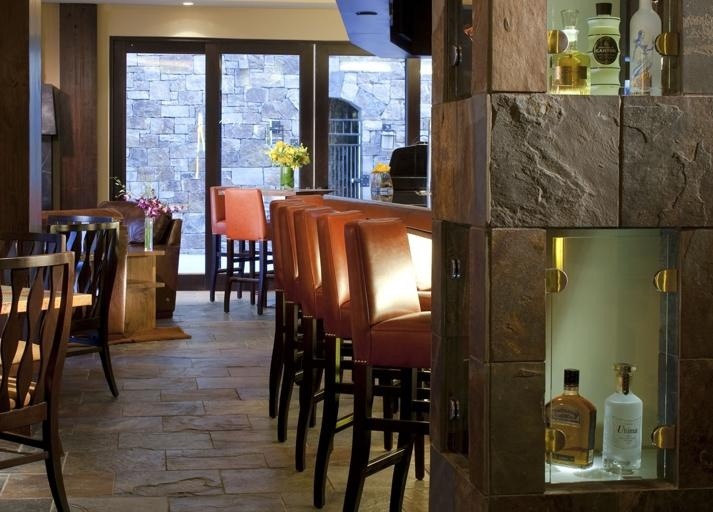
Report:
[271,140,310,185]
[111,177,186,217]
[373,163,391,173]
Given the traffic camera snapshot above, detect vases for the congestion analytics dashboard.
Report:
[144,218,154,253]
[281,166,294,188]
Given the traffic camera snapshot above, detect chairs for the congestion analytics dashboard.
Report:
[0,222,119,512]
[209,186,260,302]
[224,189,273,316]
[98,200,183,319]
[42,208,129,338]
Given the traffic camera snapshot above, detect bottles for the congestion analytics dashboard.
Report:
[627,0,663,96]
[548,6,592,96]
[582,1,621,95]
[597,362,645,475]
[544,367,599,471]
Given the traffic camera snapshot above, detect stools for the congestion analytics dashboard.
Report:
[269,198,432,512]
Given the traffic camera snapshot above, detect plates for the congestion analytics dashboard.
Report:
[296,189,334,195]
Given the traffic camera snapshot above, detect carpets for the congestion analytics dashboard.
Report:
[1,314,191,364]
